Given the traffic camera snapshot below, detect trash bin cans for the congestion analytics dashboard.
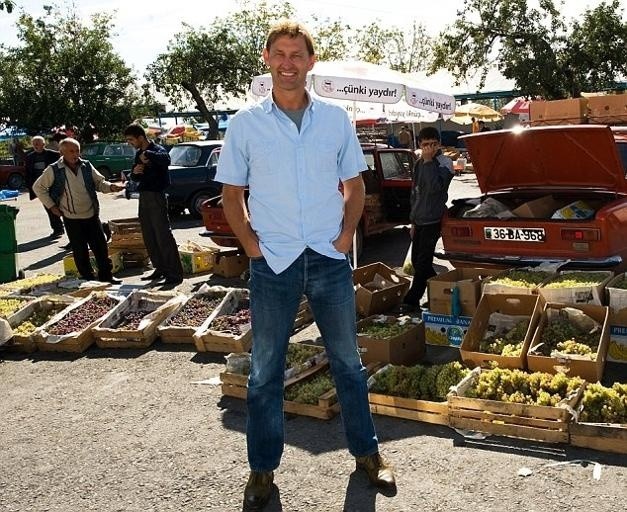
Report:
[0,204,26,284]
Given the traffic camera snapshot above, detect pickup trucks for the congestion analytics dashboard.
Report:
[0,159,26,190]
[201,142,420,254]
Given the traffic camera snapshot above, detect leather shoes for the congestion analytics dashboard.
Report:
[356,453,395,488]
[244,470,273,510]
[100,276,122,284]
[141,271,183,283]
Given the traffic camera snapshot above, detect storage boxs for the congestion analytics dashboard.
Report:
[605,271,627,324]
[538,269,615,306]
[422,310,473,349]
[424,266,505,317]
[355,314,427,366]
[512,194,557,219]
[211,249,249,278]
[480,266,557,296]
[460,292,541,370]
[352,261,411,318]
[549,199,594,220]
[528,97,586,127]
[584,93,626,125]
[63,217,150,279]
[526,304,609,382]
[607,323,627,364]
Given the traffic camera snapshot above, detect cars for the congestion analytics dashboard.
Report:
[120,139,222,220]
[438,123,627,273]
[77,141,136,182]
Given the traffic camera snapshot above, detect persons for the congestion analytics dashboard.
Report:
[119,125,185,287]
[214,23,396,508]
[399,122,414,151]
[26,136,64,237]
[9,137,27,166]
[403,127,454,307]
[32,138,125,283]
[53,133,112,251]
[471,117,491,133]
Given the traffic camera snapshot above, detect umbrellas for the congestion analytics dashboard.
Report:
[250,59,456,277]
[163,124,204,139]
[451,103,505,125]
[501,95,542,116]
